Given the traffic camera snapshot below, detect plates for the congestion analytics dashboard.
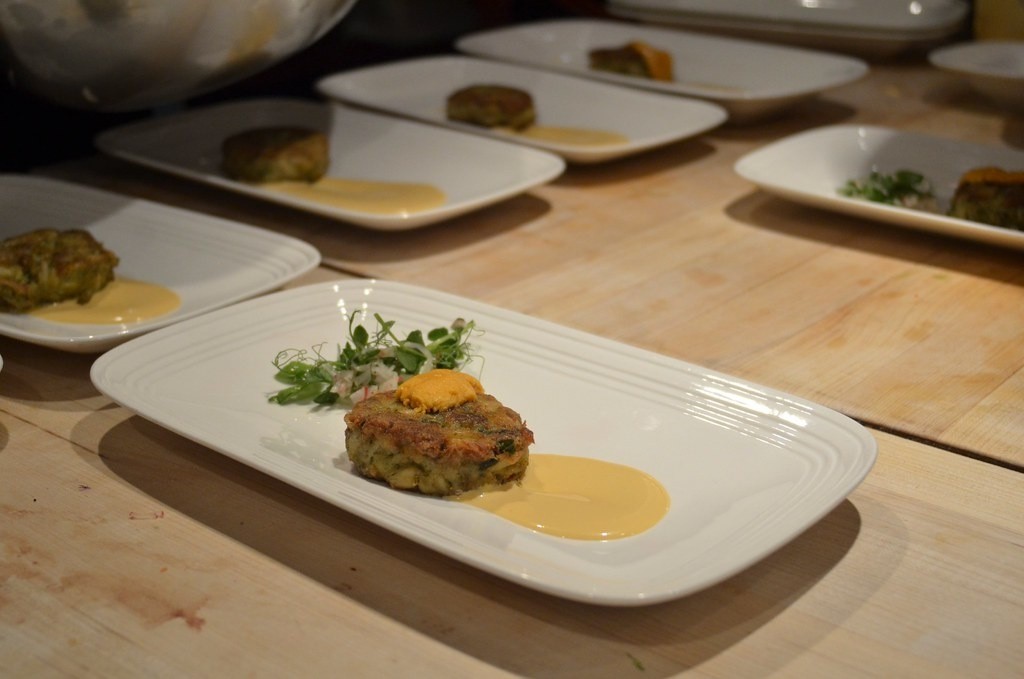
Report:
[89,279,879,609]
[95,99,566,232]
[606,0,971,63]
[0,173,322,352]
[456,15,873,125]
[733,120,1024,250]
[317,52,729,165]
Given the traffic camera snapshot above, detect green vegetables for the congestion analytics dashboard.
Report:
[266,308,486,405]
[834,166,931,206]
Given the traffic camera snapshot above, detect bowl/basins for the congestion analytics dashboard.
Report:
[927,38,1024,107]
[0,0,357,112]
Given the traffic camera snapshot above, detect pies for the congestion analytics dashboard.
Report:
[587,43,676,81]
[221,127,333,184]
[343,368,537,496]
[946,167,1024,232]
[445,84,536,130]
[0,228,121,313]
